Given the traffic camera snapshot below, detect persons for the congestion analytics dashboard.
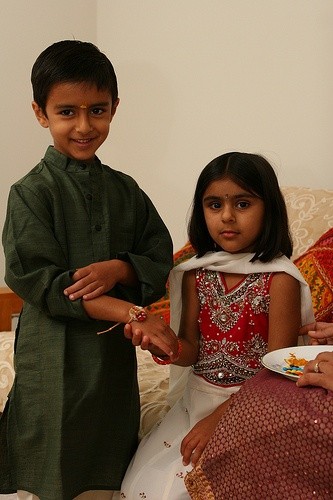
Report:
[0,39,173,500]
[118,153,333,500]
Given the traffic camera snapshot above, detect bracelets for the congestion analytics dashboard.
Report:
[152,339,182,366]
[127,305,147,325]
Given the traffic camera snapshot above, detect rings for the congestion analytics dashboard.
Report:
[314,361,320,374]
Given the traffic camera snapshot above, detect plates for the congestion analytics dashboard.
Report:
[261,344,333,381]
